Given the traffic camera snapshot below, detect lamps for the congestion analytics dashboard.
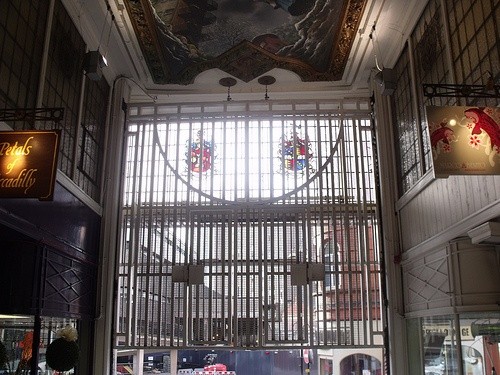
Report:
[369,26,395,97]
[83,9,113,81]
[219,77,236,102]
[258,76,276,100]
